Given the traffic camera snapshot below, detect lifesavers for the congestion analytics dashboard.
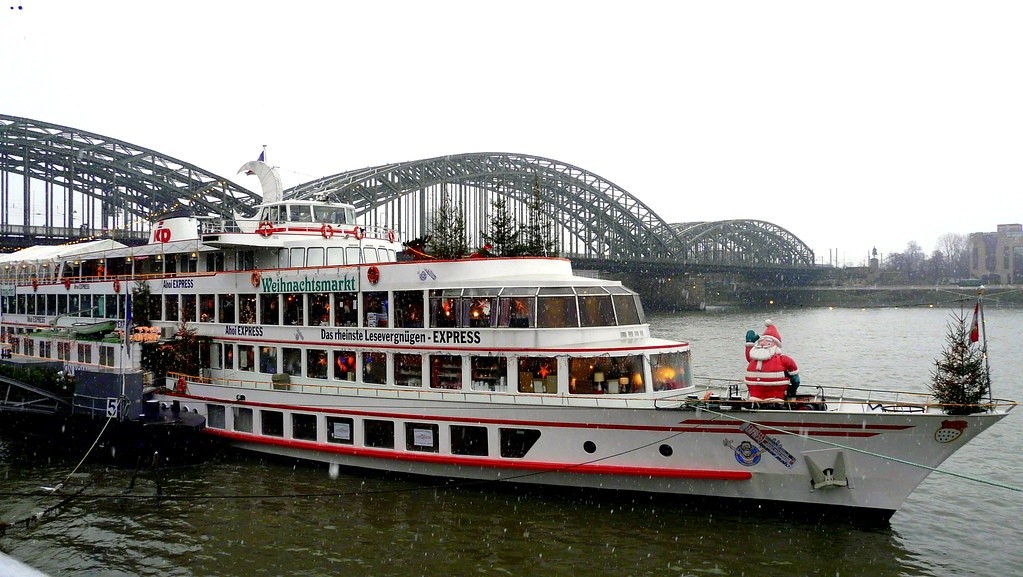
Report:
[113,280,119,292]
[354,226,364,240]
[177,378,186,393]
[250,271,261,287]
[321,224,334,239]
[33,280,37,290]
[258,221,273,237]
[389,231,395,243]
[64,278,70,289]
[367,266,380,283]
[704,392,714,400]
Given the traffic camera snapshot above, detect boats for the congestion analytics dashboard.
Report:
[71,317,113,338]
[0,200,1015,537]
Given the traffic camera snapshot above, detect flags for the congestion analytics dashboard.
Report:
[968,303,979,344]
